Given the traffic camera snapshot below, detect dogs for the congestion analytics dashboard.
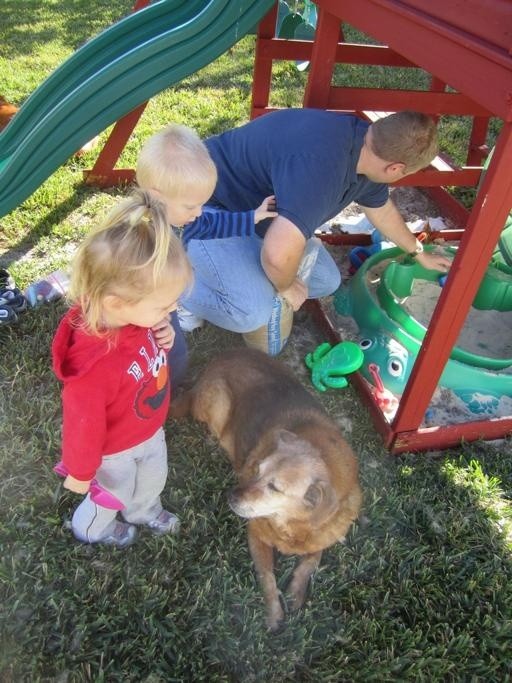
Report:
[168,346,366,635]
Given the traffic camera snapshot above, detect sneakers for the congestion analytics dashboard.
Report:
[146,508,180,533]
[176,303,204,332]
[0,269,27,324]
[108,520,136,545]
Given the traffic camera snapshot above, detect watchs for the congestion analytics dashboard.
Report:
[408,237,425,259]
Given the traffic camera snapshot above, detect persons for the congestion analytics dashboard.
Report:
[46,189,196,550]
[136,122,280,420]
[176,105,455,335]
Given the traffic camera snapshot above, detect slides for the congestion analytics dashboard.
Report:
[0,1,283,219]
